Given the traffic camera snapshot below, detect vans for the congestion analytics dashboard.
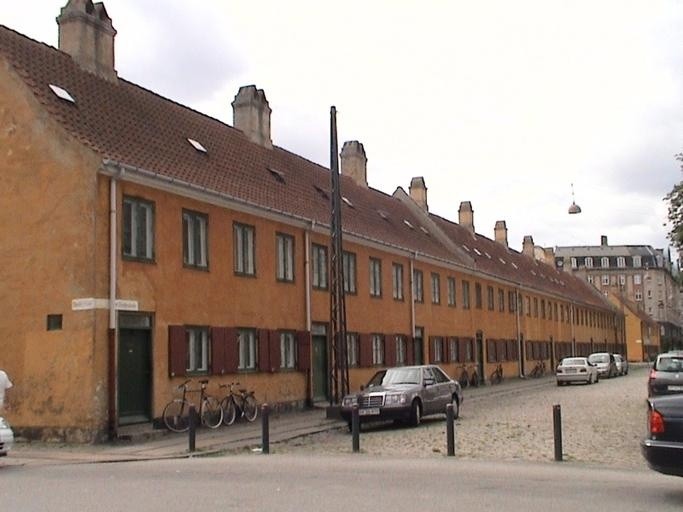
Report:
[587,351,616,379]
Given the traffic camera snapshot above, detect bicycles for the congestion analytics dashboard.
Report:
[162,377,223,433]
[458,360,547,389]
[218,381,258,426]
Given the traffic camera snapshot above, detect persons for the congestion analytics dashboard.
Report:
[0,369,13,414]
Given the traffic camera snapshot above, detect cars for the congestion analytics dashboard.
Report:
[555,355,599,386]
[647,353,683,397]
[638,391,683,478]
[611,353,628,376]
[340,365,464,431]
